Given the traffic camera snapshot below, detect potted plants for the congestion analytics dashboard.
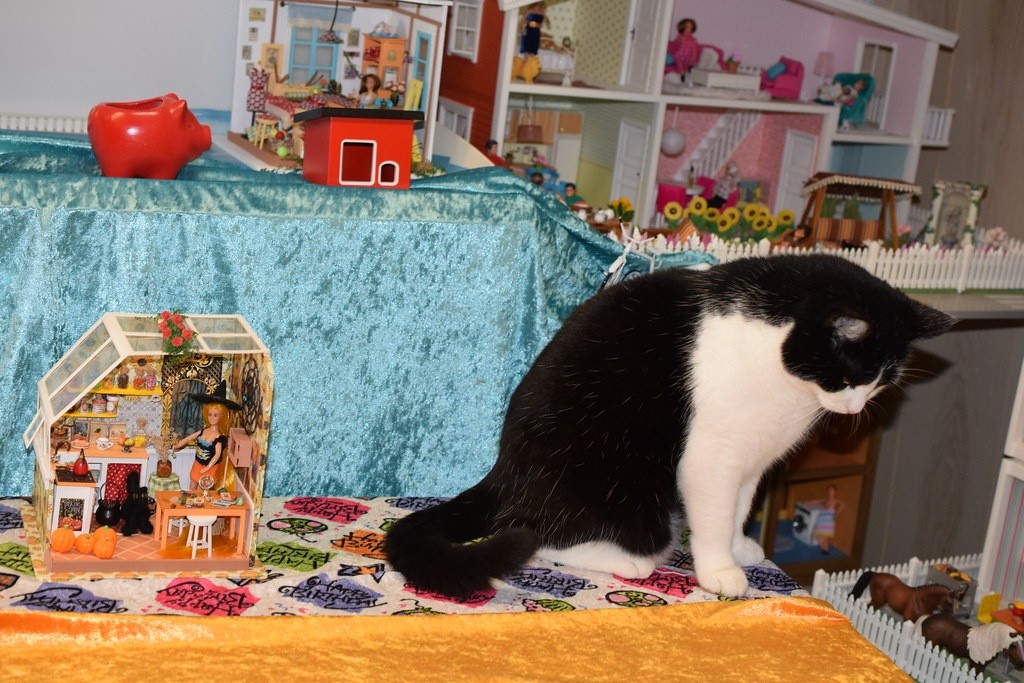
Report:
[149,427,178,477]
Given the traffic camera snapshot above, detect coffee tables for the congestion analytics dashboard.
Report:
[690,67,761,96]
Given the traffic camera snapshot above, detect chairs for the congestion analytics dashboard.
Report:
[654,175,739,229]
[978,593,1003,625]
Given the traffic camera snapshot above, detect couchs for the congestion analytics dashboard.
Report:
[664,40,804,99]
[814,72,876,128]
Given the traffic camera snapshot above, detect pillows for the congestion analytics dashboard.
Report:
[766,63,785,80]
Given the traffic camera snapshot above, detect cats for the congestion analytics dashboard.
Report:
[379,254,960,599]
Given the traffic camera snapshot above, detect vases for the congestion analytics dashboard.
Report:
[727,61,739,74]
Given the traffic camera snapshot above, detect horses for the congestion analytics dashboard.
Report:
[845,567,1024,678]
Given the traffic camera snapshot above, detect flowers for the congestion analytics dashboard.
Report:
[728,49,742,65]
[157,308,199,364]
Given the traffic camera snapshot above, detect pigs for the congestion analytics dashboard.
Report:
[88,92,212,179]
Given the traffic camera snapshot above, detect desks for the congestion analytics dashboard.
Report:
[153,490,250,555]
[989,609,1024,676]
[654,179,704,195]
[148,470,181,503]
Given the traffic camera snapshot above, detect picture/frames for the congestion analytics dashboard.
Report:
[261,43,284,71]
[62,420,127,445]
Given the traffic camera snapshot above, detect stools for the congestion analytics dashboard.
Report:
[168,516,189,538]
[253,114,279,151]
[186,515,217,559]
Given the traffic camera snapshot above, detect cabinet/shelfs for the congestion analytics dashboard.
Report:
[62,384,163,418]
[362,30,406,87]
[760,420,882,586]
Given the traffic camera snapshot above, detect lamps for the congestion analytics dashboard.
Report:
[314,0,342,43]
[813,52,834,84]
[513,95,545,144]
[657,104,687,157]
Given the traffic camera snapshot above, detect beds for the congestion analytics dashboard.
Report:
[514,36,579,86]
